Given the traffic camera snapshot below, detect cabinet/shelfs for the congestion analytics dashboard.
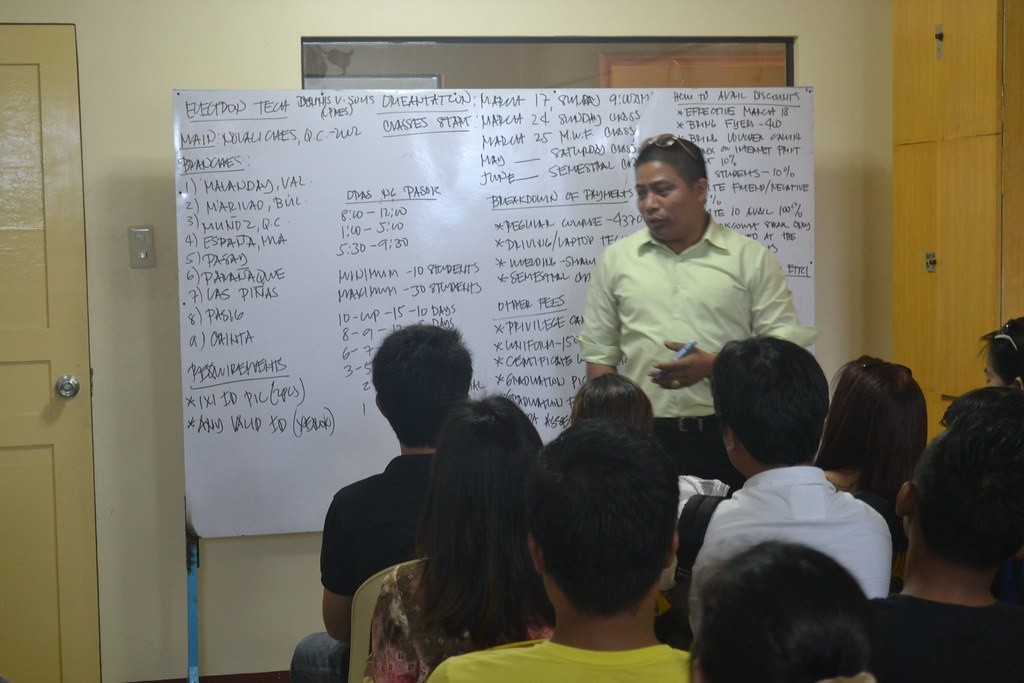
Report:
[891,0,1023,446]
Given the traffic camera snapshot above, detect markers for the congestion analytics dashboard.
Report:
[653,338,698,383]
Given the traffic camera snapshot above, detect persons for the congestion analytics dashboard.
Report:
[364,398,557,683]
[289,327,473,682]
[813,355,927,492]
[939,387,1024,423]
[573,373,653,434]
[685,541,875,682]
[865,396,1022,682]
[424,420,692,682]
[984,317,1024,391]
[575,134,820,485]
[689,337,893,638]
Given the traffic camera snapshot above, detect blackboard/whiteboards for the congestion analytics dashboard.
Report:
[172,85,818,539]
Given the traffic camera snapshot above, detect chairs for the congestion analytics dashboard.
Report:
[346,557,422,682]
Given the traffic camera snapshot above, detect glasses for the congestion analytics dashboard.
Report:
[858,359,913,378]
[993,317,1020,353]
[638,133,697,161]
